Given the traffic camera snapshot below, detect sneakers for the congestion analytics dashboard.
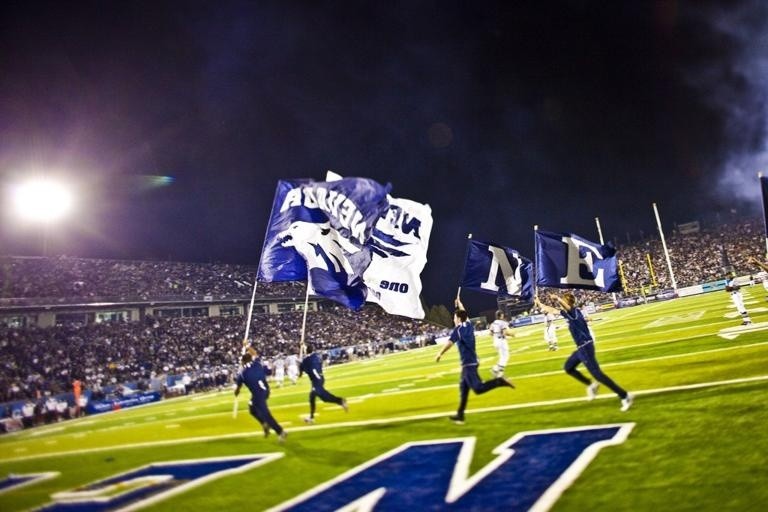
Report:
[449,415,465,425]
[586,382,600,402]
[620,393,636,412]
[500,376,516,388]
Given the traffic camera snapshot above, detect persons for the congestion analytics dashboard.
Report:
[540,309,558,351]
[511,216,768,326]
[489,309,515,379]
[435,298,515,425]
[1,255,456,443]
[534,293,634,411]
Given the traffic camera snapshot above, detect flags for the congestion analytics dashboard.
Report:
[458,241,535,302]
[255,178,392,313]
[306,170,433,321]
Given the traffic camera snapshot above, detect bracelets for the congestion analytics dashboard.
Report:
[438,354,442,358]
